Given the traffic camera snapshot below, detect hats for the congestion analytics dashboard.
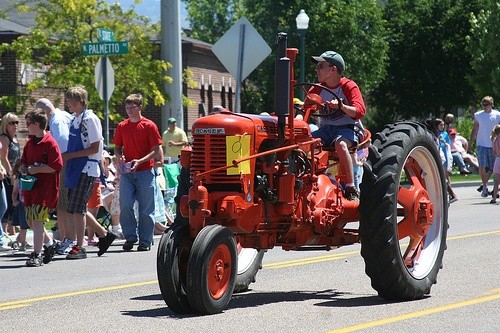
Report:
[167,117,177,123]
[448,128,457,134]
[311,51,346,73]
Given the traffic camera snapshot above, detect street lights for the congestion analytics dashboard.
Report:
[295,8,311,102]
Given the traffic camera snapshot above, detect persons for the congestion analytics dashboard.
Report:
[468,96,500,203]
[351,139,372,192]
[422,114,479,202]
[303,51,366,201]
[213,98,319,133]
[162,118,188,158]
[0,85,175,267]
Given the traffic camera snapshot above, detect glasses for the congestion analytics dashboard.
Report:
[8,120,19,126]
[482,104,489,106]
[315,64,333,70]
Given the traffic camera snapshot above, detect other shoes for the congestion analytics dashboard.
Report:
[26,244,34,249]
[122,238,138,250]
[345,186,360,201]
[0,245,11,251]
[19,245,26,251]
[57,240,78,255]
[460,169,472,174]
[43,240,61,265]
[96,232,116,257]
[137,243,154,251]
[65,245,87,259]
[476,184,500,203]
[25,251,44,267]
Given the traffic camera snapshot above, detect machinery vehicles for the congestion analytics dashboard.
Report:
[156,30,450,316]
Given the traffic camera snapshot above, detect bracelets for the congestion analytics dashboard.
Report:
[341,103,344,109]
